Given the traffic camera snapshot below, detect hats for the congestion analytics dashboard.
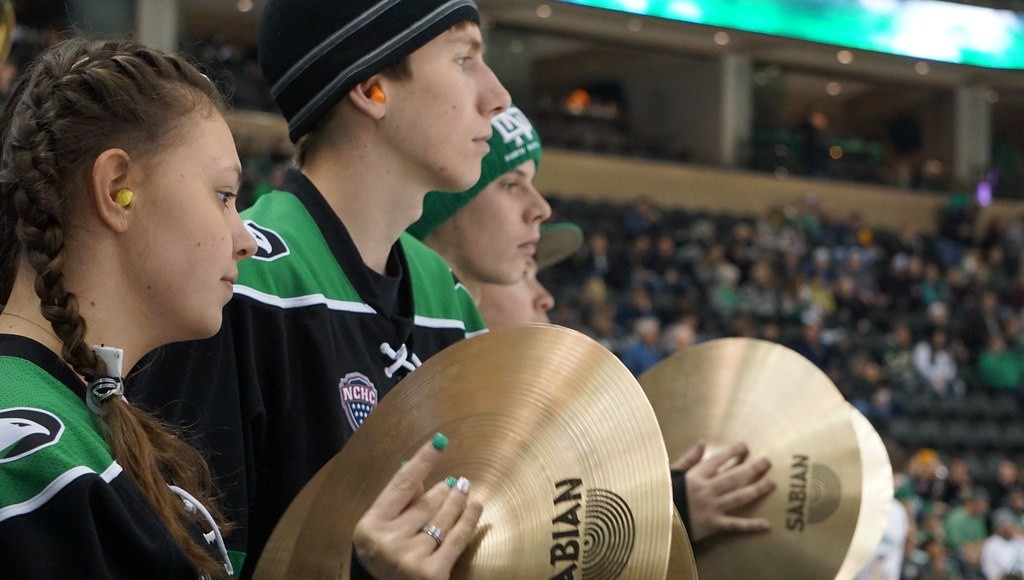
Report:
[538,223,583,268]
[259,0,480,145]
[405,105,542,240]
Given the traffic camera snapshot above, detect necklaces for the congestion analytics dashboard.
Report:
[3,311,63,345]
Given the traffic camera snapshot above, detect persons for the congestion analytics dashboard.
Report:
[533,195,1024,580]
[0,1,773,580]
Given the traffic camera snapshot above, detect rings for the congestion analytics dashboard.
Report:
[423,524,442,545]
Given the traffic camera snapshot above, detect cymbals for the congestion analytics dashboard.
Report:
[834,395,894,579]
[283,322,674,579]
[248,449,699,580]
[637,337,863,580]
